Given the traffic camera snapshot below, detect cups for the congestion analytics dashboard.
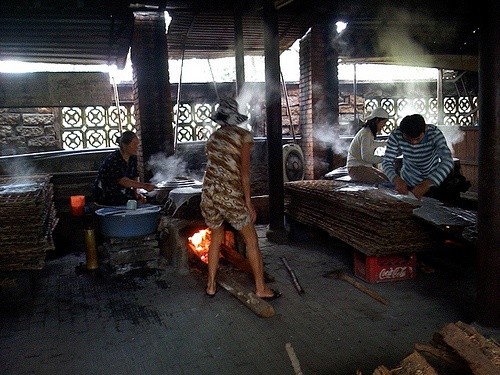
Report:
[127,200,138,210]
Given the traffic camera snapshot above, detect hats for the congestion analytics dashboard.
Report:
[364,108,388,120]
[209,97,248,126]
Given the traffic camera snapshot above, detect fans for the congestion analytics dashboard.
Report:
[283,144,305,183]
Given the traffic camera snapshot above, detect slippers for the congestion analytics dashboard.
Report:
[261,289,282,300]
[205,285,217,296]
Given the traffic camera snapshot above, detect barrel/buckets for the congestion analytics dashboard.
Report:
[70,195,86,215]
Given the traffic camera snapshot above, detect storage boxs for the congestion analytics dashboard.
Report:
[353,250,417,284]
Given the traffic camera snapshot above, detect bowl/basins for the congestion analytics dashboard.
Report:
[94,206,162,236]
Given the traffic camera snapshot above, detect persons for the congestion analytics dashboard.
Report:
[199,96,282,302]
[92,131,160,206]
[382,114,455,200]
[346,107,392,188]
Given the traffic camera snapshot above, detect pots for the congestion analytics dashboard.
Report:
[169,188,203,219]
